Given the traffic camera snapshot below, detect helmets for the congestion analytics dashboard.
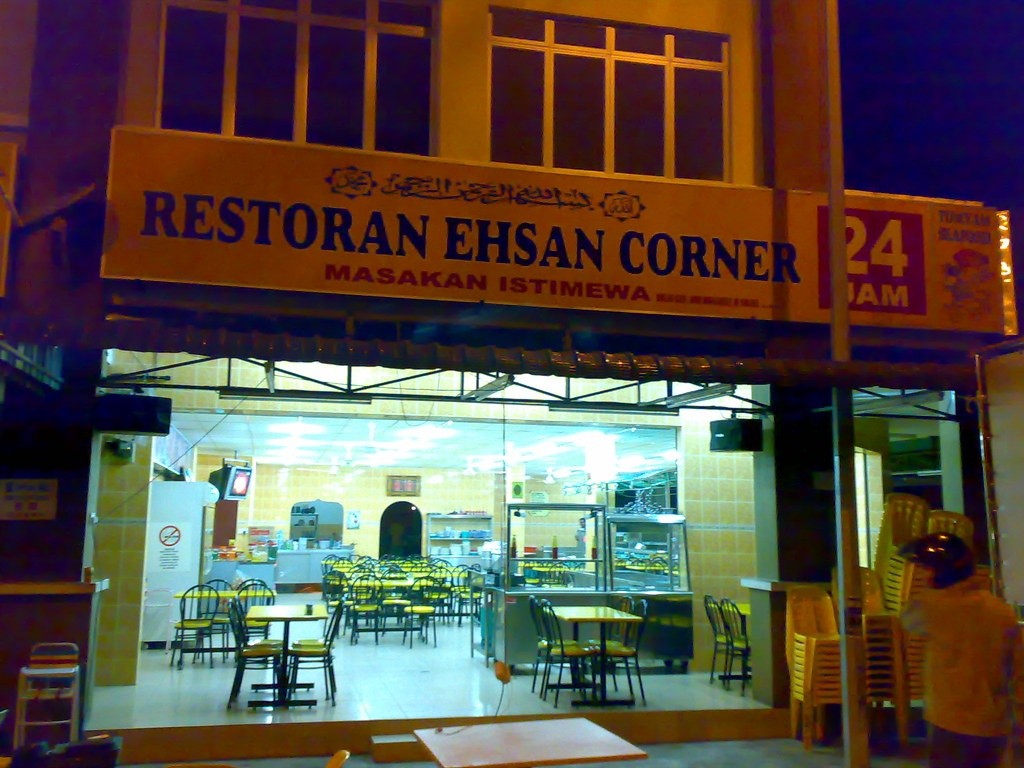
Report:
[896,533,975,587]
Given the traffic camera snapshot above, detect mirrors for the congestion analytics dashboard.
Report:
[290,499,344,547]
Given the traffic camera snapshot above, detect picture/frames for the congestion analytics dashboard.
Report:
[386,475,423,497]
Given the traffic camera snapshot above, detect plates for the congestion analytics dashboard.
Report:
[429,541,471,555]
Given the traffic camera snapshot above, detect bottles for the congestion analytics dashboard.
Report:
[591,536,598,559]
[236,527,331,564]
[511,534,516,558]
[469,529,491,538]
[552,535,558,559]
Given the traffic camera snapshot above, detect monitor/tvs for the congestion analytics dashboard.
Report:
[209,466,252,500]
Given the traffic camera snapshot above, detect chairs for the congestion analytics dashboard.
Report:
[12,644,81,752]
[616,555,680,577]
[702,594,753,696]
[327,749,351,767]
[525,595,648,712]
[171,554,490,710]
[787,494,991,749]
[522,558,573,587]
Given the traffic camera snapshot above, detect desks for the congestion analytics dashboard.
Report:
[710,603,752,672]
[535,566,580,584]
[245,606,329,709]
[540,607,642,705]
[332,566,463,571]
[174,591,277,671]
[415,717,651,768]
[520,563,563,568]
[626,564,667,572]
[331,571,469,579]
[331,580,433,589]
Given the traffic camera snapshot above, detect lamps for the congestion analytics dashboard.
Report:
[218,387,374,405]
[547,402,680,417]
[666,385,737,410]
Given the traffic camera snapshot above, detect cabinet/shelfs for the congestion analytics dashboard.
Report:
[293,512,319,540]
[427,511,494,568]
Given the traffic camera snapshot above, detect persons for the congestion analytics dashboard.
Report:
[896,532,1024,768]
[575,518,586,555]
[389,516,404,557]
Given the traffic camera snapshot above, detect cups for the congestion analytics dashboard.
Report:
[212,539,236,561]
[437,526,469,538]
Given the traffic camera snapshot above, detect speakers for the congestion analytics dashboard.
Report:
[94,394,172,437]
[710,419,763,452]
